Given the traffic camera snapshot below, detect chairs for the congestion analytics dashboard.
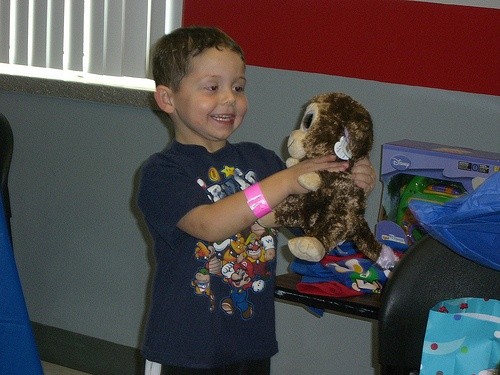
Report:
[371,229,500,375]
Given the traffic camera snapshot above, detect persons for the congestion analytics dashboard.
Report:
[137,25,375,375]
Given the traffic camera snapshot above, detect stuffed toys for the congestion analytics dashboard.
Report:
[252,91,395,268]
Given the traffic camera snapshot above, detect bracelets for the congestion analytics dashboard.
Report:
[241,184,271,220]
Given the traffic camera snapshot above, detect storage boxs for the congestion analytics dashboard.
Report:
[374,138,500,251]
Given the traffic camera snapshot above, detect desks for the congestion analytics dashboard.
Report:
[274,273,379,320]
